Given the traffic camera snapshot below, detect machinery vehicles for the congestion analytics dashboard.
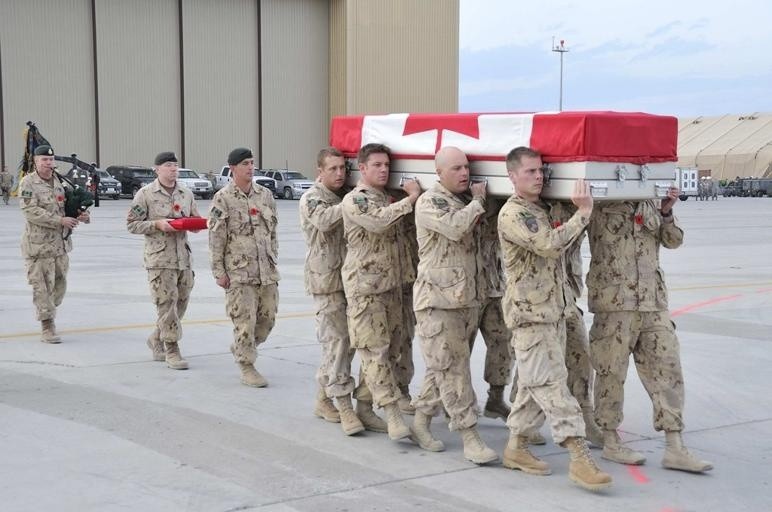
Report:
[675,167,772,201]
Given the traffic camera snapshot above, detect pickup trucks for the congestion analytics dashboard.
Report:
[199,165,278,199]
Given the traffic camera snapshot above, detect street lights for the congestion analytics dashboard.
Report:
[550,34,570,111]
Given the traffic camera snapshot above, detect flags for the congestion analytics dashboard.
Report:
[8,126,51,196]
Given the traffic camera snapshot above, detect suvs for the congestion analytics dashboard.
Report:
[103,165,159,199]
[63,165,122,199]
[177,169,213,201]
[261,169,315,201]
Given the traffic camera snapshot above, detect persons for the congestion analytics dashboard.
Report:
[408,145,500,466]
[207,147,281,388]
[509,199,606,452]
[444,195,514,423]
[299,146,365,438]
[585,185,715,474]
[387,189,420,417]
[340,142,420,440]
[695,175,740,201]
[0,166,14,205]
[125,152,203,370]
[18,144,91,344]
[496,146,614,490]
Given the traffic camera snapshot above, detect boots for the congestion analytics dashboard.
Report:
[239,362,267,387]
[664,430,715,471]
[315,385,445,451]
[563,408,645,492]
[147,330,166,360]
[40,320,61,345]
[503,430,550,475]
[166,343,189,369]
[484,386,511,422]
[462,424,499,463]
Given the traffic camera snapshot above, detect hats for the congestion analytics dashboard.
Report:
[228,148,252,165]
[154,152,177,165]
[34,145,54,156]
